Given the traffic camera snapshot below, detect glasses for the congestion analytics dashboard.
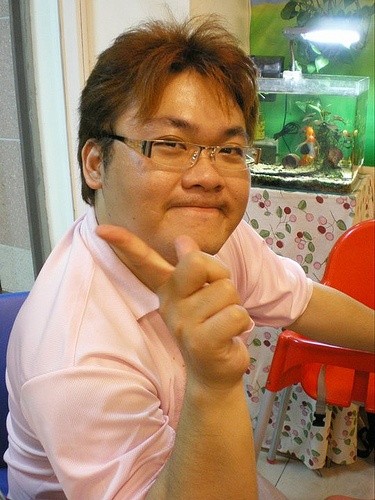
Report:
[97,134,258,173]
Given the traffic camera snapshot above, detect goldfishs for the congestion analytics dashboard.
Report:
[324,146,342,170]
[295,126,318,154]
[297,153,315,166]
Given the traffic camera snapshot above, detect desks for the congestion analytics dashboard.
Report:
[240,166,375,459]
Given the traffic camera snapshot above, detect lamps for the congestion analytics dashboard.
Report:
[282,24,365,49]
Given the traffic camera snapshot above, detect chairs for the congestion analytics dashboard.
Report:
[254,220,375,465]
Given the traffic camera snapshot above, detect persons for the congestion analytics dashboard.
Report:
[3,1,375,500]
